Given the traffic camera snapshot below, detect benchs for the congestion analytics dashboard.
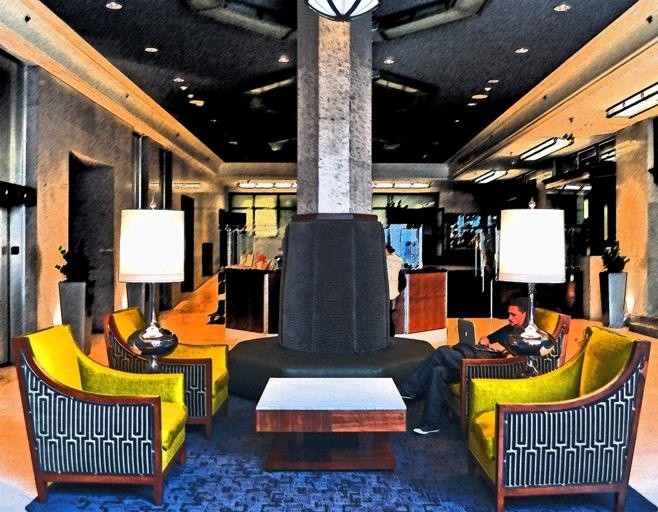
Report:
[227,336,436,403]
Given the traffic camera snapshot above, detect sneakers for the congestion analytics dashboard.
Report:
[413,424,440,434]
[398,388,411,399]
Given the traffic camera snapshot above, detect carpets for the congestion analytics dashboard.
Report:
[25,394,658,511]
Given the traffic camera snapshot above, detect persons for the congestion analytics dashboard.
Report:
[397,296,544,435]
[384,244,405,338]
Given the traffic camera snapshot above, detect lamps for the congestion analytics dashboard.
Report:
[118,190,185,371]
[499,196,566,378]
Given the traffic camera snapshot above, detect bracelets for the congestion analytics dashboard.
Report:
[502,351,511,358]
[499,348,507,353]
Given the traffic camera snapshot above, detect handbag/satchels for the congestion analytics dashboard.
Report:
[398,271,406,292]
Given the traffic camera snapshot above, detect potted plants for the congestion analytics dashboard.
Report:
[55,238,96,356]
[599,241,630,329]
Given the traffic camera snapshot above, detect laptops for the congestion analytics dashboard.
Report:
[458,318,496,353]
